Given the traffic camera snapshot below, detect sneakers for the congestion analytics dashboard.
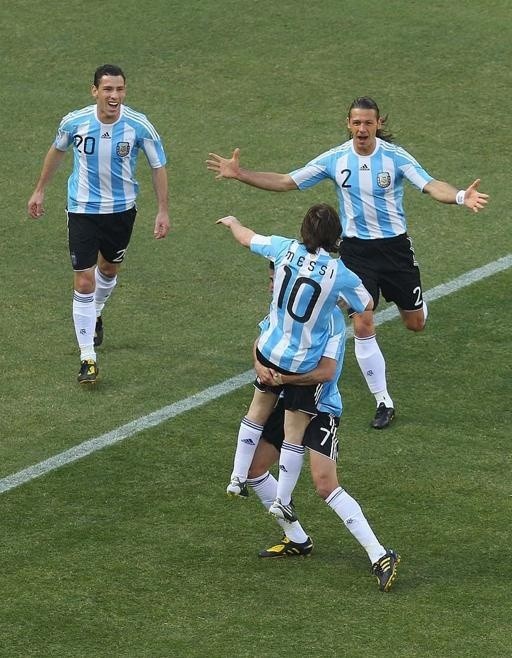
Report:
[372,549,401,592]
[93,316,103,347]
[370,401,397,430]
[77,359,99,385]
[226,477,249,500]
[258,531,315,560]
[269,498,299,524]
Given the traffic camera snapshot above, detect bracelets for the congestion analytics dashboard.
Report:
[455,190,465,206]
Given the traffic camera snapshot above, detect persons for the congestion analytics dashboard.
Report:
[204,95,490,429]
[27,64,171,384]
[246,261,402,592]
[214,203,375,523]
